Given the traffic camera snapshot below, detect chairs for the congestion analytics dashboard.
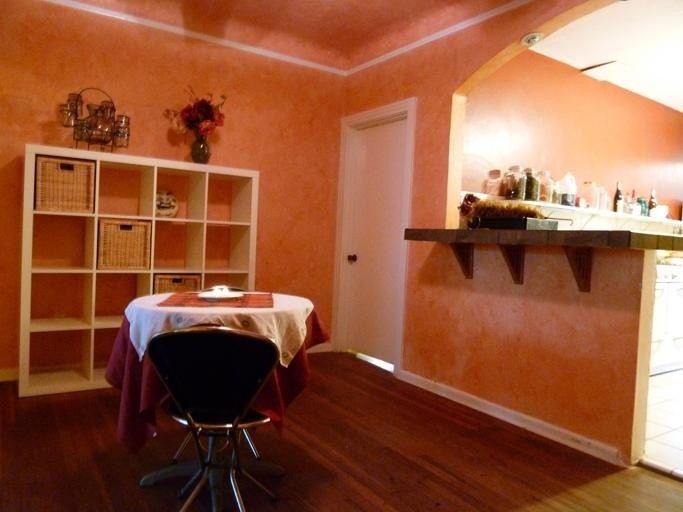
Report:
[146,328,282,512]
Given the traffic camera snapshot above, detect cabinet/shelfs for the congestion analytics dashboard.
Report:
[16,142,260,400]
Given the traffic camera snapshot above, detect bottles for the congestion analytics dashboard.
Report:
[479,165,656,217]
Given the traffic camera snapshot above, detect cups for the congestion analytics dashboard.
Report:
[215,286,228,296]
[60,93,130,147]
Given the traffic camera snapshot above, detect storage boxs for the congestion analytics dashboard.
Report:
[34,153,95,213]
[97,218,152,272]
[153,275,202,295]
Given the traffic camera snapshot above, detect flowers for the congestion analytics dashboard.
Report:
[162,83,228,145]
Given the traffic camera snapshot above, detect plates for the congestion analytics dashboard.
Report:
[198,292,243,300]
[156,189,179,218]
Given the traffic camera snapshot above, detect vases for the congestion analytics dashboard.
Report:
[191,136,210,164]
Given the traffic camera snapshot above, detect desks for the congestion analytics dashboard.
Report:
[131,291,315,463]
